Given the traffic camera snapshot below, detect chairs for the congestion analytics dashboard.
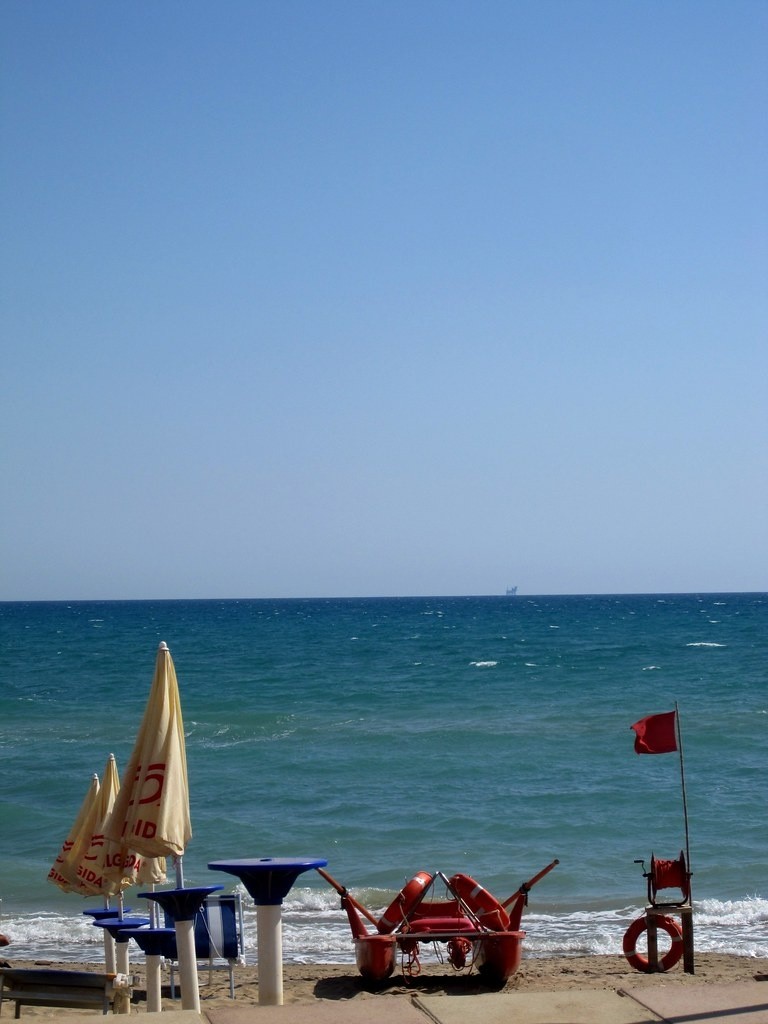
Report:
[167,893,245,1001]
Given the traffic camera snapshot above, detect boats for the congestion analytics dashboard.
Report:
[315,859,561,985]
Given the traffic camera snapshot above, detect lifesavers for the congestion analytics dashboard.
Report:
[623,916,683,972]
[379,871,509,934]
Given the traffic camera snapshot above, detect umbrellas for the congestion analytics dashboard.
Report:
[101,640,193,859]
[46,753,168,898]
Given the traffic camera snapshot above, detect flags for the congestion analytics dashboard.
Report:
[630,711,678,754]
